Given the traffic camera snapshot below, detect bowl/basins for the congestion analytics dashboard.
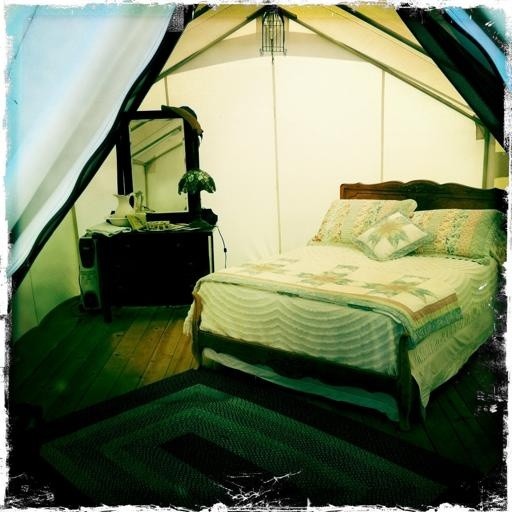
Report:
[106,217,129,227]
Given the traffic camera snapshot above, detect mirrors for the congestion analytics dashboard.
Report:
[117,105,201,222]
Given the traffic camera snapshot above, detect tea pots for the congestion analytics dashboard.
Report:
[111,192,136,219]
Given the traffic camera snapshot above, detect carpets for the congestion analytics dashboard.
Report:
[8,364,505,512]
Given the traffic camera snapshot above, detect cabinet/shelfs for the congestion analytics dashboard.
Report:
[96,213,218,323]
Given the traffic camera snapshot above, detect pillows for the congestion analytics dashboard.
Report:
[411,208,507,265]
[355,213,433,261]
[309,196,418,252]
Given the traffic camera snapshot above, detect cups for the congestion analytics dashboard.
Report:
[145,221,171,230]
[135,213,146,227]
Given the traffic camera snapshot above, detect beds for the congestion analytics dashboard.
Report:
[183,175,507,431]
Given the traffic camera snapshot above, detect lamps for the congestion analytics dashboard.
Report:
[257,9,289,58]
[175,167,216,216]
